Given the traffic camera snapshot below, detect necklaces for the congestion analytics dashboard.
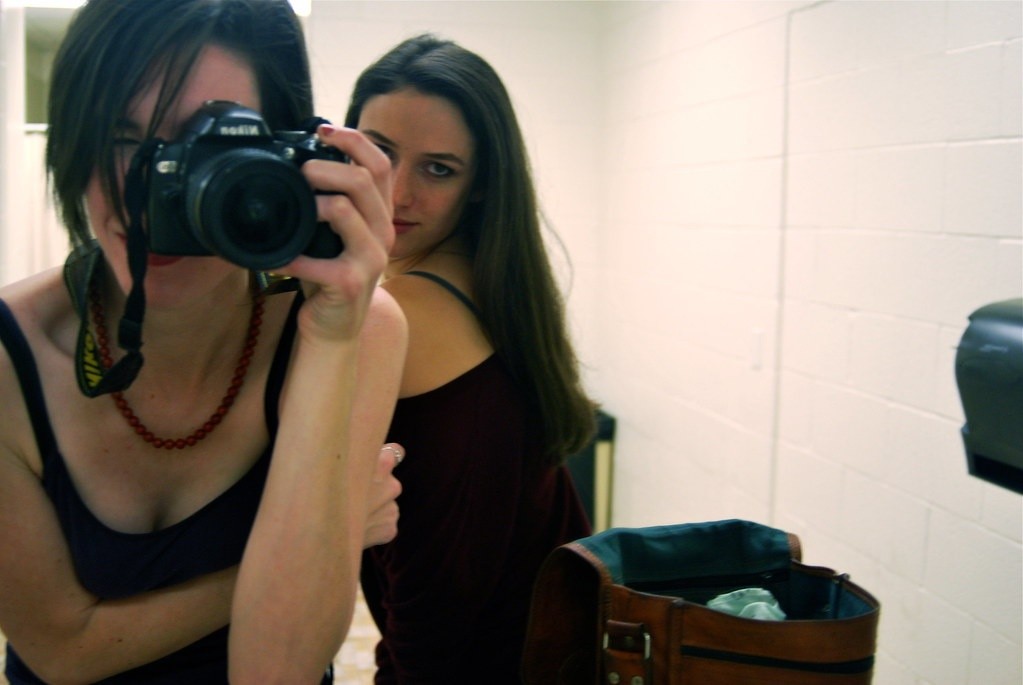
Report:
[92,268,266,448]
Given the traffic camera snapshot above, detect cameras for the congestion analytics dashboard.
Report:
[143,98,352,272]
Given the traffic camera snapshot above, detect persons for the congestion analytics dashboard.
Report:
[343,36,598,685]
[0,0,407,685]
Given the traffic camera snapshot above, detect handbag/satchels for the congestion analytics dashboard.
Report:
[519,518,880,685]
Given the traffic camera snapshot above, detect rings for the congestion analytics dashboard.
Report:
[382,447,402,464]
